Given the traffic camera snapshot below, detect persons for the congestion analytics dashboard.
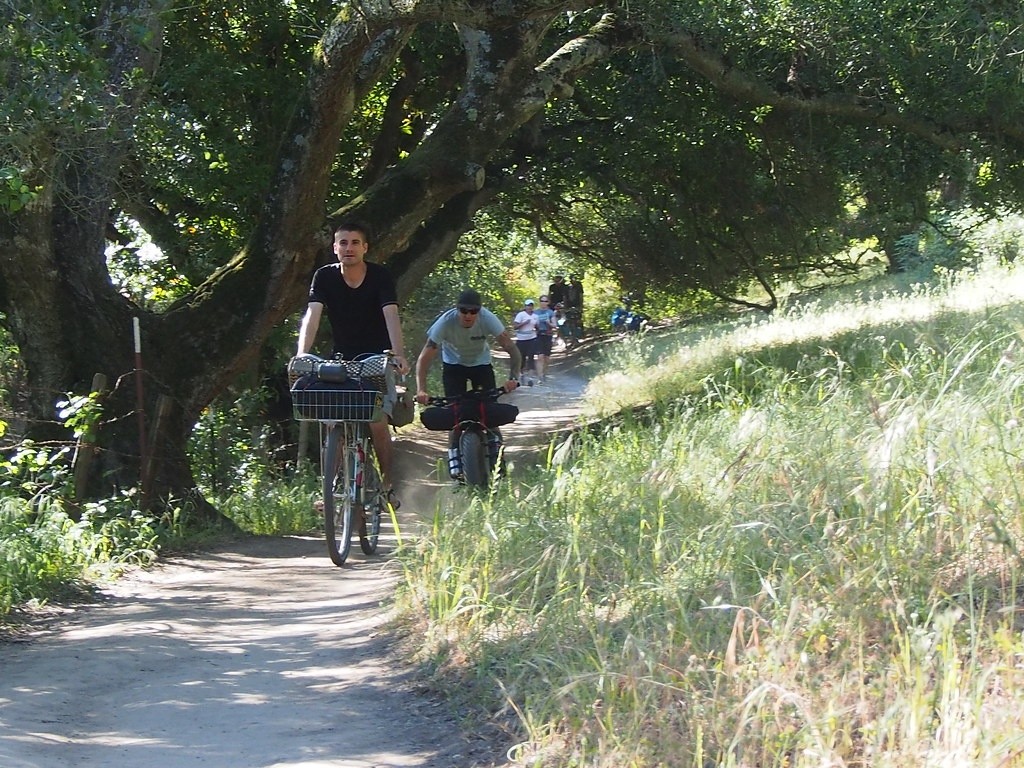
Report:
[514,300,540,380]
[297,224,409,512]
[532,295,557,384]
[416,289,522,494]
[549,274,584,349]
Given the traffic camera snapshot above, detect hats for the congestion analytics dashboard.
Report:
[455,289,482,309]
[525,299,534,306]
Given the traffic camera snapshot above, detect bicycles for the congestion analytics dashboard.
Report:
[289,351,403,569]
[554,307,579,355]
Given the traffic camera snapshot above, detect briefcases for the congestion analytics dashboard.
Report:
[286,355,391,395]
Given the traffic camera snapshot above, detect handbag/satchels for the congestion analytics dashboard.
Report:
[388,385,415,428]
[419,393,519,432]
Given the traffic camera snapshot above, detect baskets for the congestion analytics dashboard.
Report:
[289,376,384,424]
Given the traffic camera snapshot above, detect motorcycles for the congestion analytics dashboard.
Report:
[415,382,520,501]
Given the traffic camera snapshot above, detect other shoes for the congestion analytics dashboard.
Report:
[537,378,544,386]
[380,486,401,513]
[520,374,533,383]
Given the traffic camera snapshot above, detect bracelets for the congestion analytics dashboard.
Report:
[509,378,518,380]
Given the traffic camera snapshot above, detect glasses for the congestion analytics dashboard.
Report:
[459,307,479,315]
[540,300,549,302]
